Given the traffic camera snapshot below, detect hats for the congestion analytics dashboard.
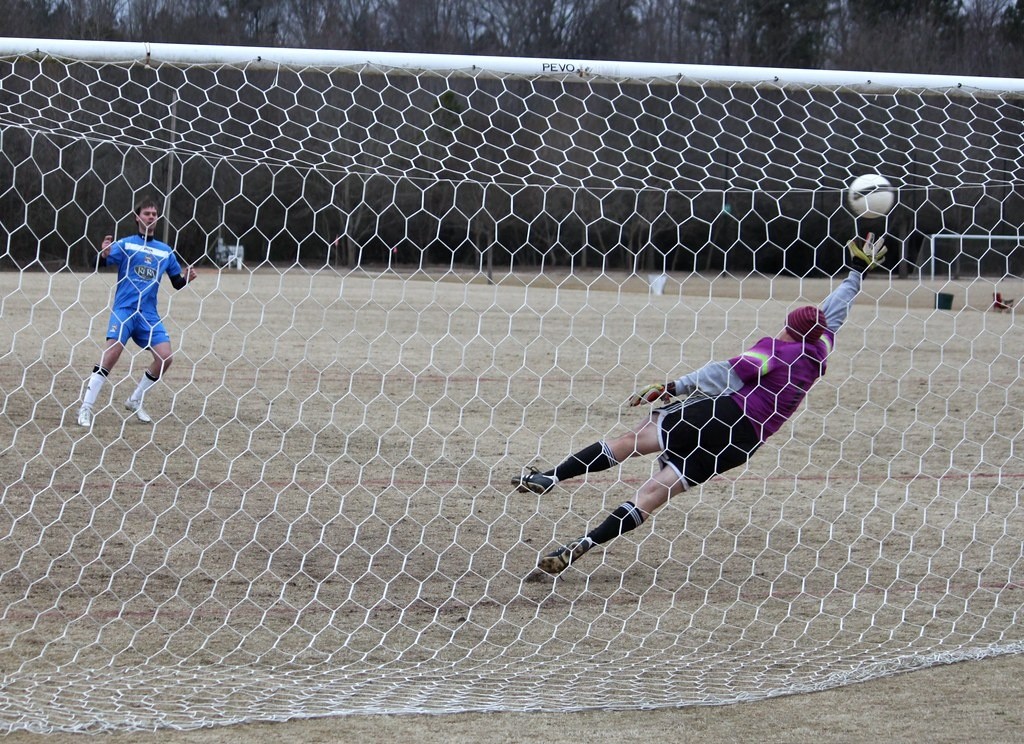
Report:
[784,305,827,344]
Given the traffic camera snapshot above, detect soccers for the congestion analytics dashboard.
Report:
[847,174,895,219]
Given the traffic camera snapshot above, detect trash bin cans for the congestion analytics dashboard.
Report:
[935,292,955,310]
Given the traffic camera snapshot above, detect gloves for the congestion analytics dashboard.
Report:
[847,232,887,279]
[628,384,671,407]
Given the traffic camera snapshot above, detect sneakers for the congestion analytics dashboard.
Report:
[125,395,151,423]
[537,538,589,582]
[76,405,91,426]
[510,466,555,495]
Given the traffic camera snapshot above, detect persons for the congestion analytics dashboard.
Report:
[76,196,198,428]
[509,232,887,574]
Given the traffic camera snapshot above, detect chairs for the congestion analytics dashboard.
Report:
[993,293,1014,313]
